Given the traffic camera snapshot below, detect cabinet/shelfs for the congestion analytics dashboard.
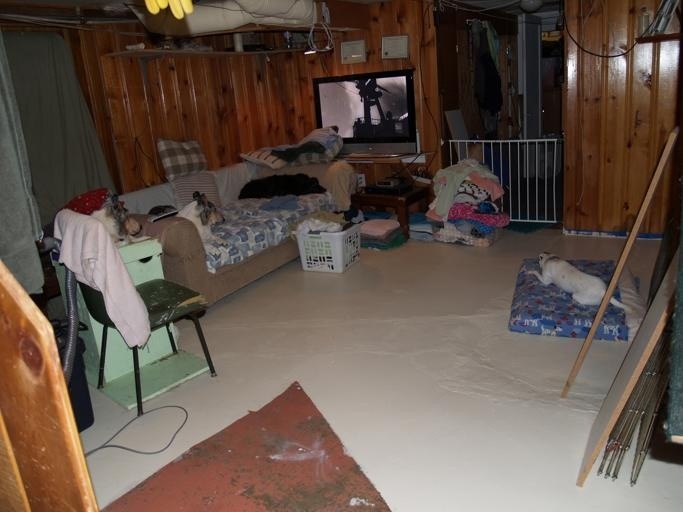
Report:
[47,236,180,384]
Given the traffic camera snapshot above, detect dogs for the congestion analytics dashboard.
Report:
[524,251,636,313]
[146,190,230,256]
[84,191,142,246]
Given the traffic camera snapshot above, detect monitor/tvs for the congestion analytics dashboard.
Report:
[312,69,417,155]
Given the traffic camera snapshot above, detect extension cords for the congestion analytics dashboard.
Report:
[412,175,432,184]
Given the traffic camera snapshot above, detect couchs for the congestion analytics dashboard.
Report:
[113,159,358,320]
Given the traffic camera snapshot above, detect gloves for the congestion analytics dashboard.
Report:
[144,0,194,19]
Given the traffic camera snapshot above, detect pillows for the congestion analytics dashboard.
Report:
[155,137,222,212]
[238,134,343,170]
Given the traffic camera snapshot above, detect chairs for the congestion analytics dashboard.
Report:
[53,207,217,417]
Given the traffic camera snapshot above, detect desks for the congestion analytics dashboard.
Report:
[352,187,430,239]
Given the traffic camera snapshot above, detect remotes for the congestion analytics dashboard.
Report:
[147,209,178,223]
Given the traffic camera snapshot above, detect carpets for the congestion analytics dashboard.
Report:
[100,380,394,512]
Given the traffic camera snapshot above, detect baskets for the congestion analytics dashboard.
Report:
[296,223,361,273]
